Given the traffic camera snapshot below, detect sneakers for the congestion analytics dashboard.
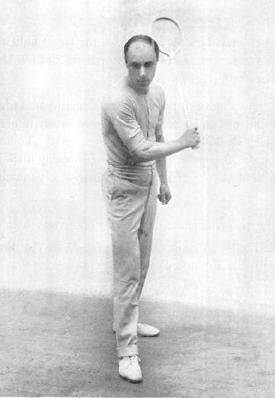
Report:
[118,355,143,384]
[112,322,160,339]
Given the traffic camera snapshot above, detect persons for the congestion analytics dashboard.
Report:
[101,34,201,384]
